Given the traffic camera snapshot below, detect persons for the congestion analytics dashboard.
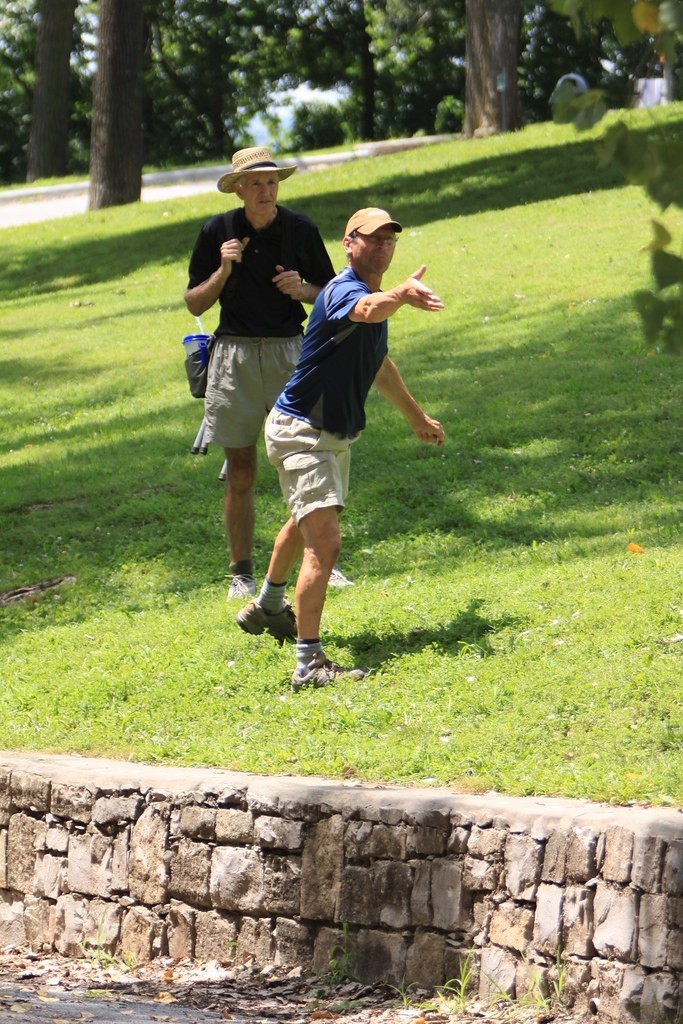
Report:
[185,147,353,597]
[236,207,445,691]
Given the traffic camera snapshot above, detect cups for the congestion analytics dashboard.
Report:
[182,335,209,357]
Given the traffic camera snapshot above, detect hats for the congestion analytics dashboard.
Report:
[345,207,402,236]
[217,146,297,193]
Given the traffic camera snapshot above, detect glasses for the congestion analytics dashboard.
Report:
[349,235,399,242]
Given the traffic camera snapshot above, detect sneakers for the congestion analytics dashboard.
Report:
[291,651,364,694]
[226,574,259,602]
[236,595,298,636]
[327,569,355,589]
